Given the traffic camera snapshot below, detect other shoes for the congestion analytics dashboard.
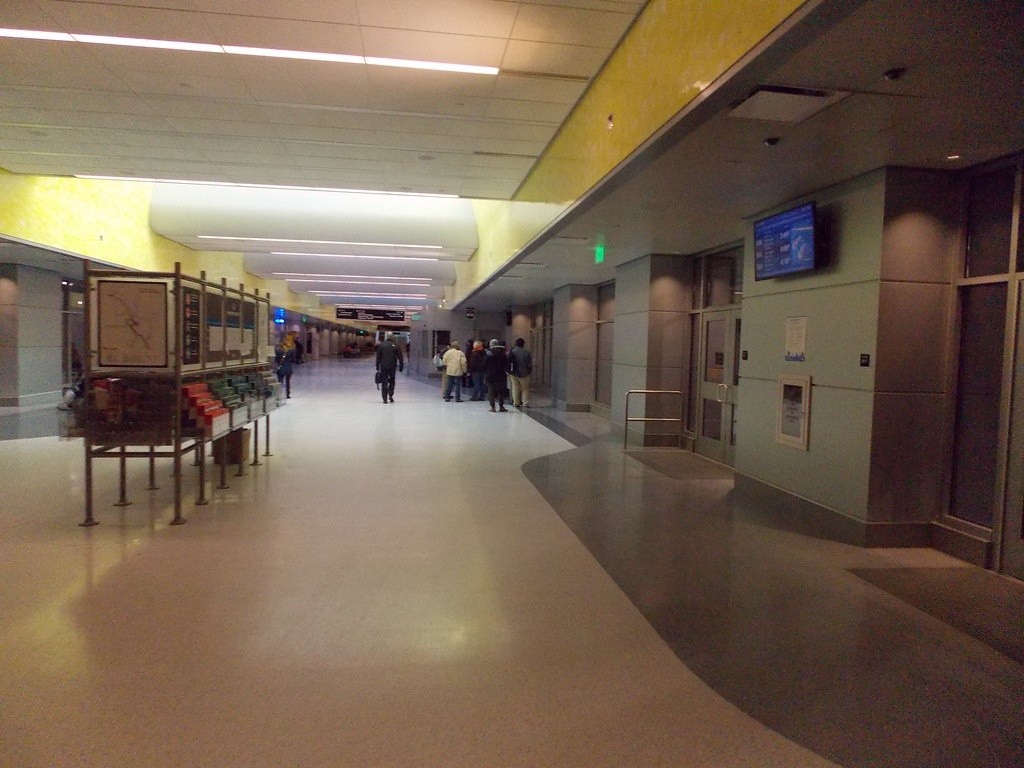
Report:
[479,398,484,400]
[389,396,393,402]
[489,408,495,412]
[445,400,450,402]
[456,399,462,402]
[444,395,453,398]
[383,399,386,403]
[499,408,507,412]
[470,398,477,401]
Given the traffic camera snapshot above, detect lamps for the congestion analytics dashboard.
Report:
[464,306,477,321]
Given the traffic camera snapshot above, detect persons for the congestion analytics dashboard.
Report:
[293,334,306,364]
[505,337,533,408]
[465,339,474,388]
[273,348,296,399]
[485,338,508,413]
[469,339,491,401]
[432,340,468,402]
[480,338,490,349]
[73,342,81,364]
[375,337,404,404]
[344,343,352,359]
[405,342,410,359]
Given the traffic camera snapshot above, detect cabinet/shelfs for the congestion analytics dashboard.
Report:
[81,259,275,526]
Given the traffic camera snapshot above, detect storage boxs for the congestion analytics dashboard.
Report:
[248,373,261,382]
[214,428,252,465]
[182,383,208,410]
[189,392,212,419]
[228,402,248,427]
[263,376,275,385]
[242,390,258,404]
[257,386,273,400]
[257,370,272,379]
[234,382,250,393]
[205,408,229,437]
[247,398,264,419]
[196,399,223,429]
[204,377,228,391]
[229,374,246,386]
[221,394,241,408]
[212,386,234,398]
[267,381,282,391]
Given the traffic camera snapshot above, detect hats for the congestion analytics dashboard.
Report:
[490,339,499,347]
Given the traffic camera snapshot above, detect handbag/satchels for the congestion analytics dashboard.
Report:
[375,370,382,390]
[505,350,517,376]
[433,353,444,367]
[276,356,287,375]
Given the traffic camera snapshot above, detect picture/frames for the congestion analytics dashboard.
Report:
[774,373,813,454]
[96,279,170,368]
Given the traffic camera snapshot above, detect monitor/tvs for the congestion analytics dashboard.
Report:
[754,201,818,280]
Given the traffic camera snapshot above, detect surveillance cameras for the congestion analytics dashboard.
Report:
[885,69,904,82]
[763,137,780,147]
